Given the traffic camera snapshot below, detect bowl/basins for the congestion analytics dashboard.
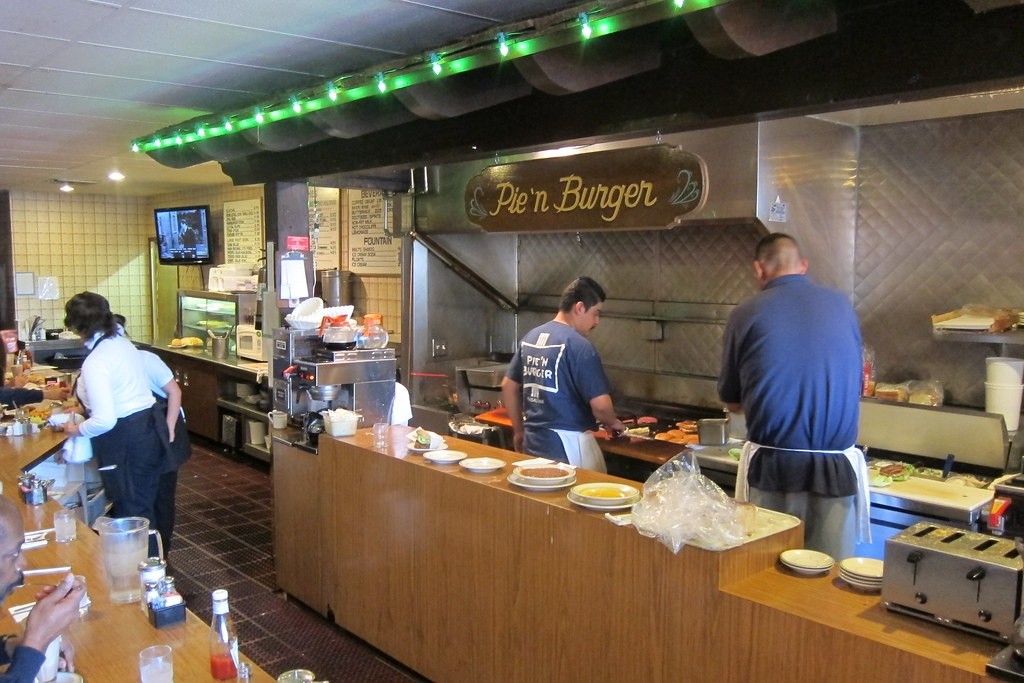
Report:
[283,297,354,331]
[512,465,577,485]
[321,410,363,436]
[570,483,639,505]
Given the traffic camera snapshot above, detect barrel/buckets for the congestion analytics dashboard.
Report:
[321,267,354,306]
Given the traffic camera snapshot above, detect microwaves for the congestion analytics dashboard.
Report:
[236,324,268,362]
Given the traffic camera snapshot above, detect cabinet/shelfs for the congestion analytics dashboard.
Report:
[151,348,220,444]
[176,288,258,351]
[215,364,273,464]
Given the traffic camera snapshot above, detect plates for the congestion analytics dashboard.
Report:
[566,492,642,511]
[406,441,448,455]
[34,671,83,683]
[422,449,468,465]
[506,473,577,491]
[459,457,506,473]
[779,548,884,592]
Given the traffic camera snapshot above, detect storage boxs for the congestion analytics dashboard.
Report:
[697,417,729,446]
[222,414,242,448]
[217,274,259,295]
[206,266,252,293]
[148,601,187,628]
[324,415,359,438]
[86,487,106,523]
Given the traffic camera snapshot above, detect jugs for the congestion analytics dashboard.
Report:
[306,412,324,446]
[98,516,163,603]
[319,314,356,352]
[356,314,389,349]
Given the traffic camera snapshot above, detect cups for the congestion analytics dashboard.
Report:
[212,337,229,358]
[138,644,173,683]
[54,510,78,542]
[34,636,59,683]
[983,357,1024,432]
[267,411,287,429]
[277,669,316,683]
[10,365,23,377]
[373,422,389,448]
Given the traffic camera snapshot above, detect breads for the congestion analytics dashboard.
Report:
[867,381,939,407]
[171,337,203,346]
[655,429,700,445]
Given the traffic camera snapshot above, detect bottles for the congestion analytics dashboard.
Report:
[31,479,44,505]
[22,417,32,435]
[31,332,36,341]
[208,589,239,679]
[144,582,159,618]
[137,556,167,611]
[160,576,175,596]
[60,575,88,618]
[12,417,22,436]
[39,328,46,340]
[22,344,34,373]
[58,375,66,389]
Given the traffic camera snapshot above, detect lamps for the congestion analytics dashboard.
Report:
[108,168,125,182]
[58,182,75,193]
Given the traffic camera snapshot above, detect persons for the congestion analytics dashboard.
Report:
[717,231,872,562]
[58,291,179,559]
[0,386,70,404]
[0,494,88,683]
[179,219,197,258]
[502,276,626,474]
[113,314,193,575]
[342,366,412,426]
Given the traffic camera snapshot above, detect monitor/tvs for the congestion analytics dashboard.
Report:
[155,205,214,265]
